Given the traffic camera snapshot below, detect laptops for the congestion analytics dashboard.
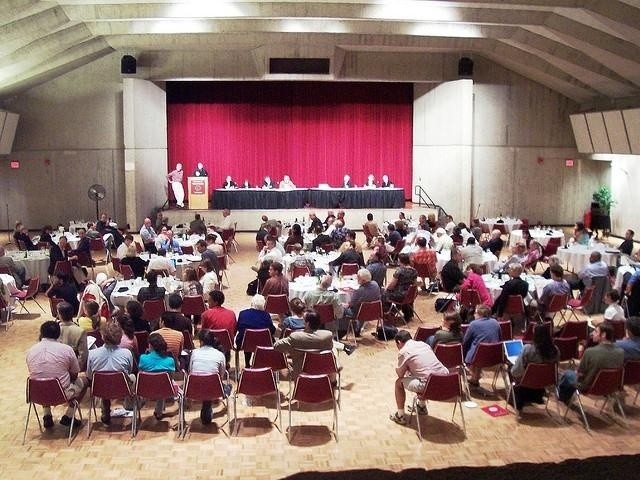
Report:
[502,339,524,365]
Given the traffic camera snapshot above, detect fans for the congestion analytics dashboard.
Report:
[88,184,105,219]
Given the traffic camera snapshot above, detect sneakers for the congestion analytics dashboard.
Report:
[408,401,429,416]
[100,414,111,428]
[388,410,407,426]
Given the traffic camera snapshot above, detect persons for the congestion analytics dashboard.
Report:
[0,212,639,429]
[193,162,207,176]
[167,163,185,208]
[222,173,395,189]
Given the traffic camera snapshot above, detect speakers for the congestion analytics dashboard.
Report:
[121,55,137,73]
[458,57,473,76]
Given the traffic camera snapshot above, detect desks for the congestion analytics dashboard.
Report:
[212,188,405,209]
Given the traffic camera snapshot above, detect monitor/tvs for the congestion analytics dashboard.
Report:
[170,259,176,268]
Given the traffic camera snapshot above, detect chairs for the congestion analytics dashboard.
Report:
[318,183,332,188]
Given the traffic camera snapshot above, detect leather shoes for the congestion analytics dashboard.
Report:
[58,413,81,427]
[345,343,356,355]
[332,380,339,390]
[43,414,56,429]
